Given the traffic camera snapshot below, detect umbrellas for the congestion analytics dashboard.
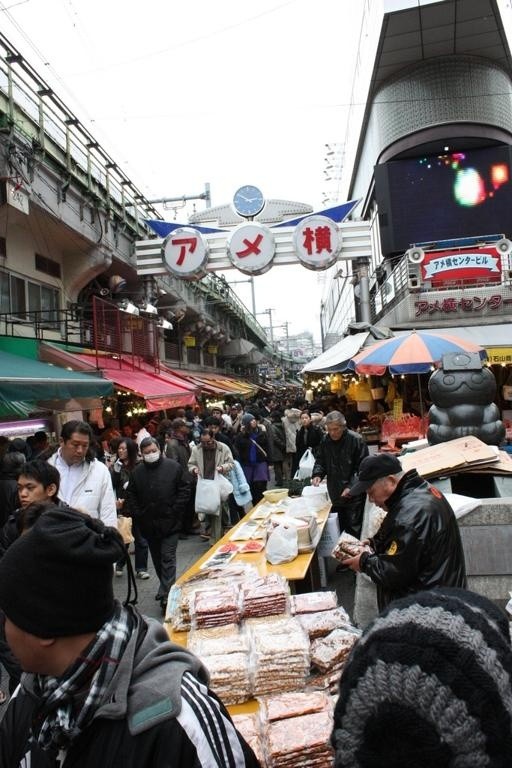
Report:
[348,328,483,439]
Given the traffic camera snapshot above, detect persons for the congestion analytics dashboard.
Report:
[312,410,370,573]
[1,430,67,555]
[340,455,470,616]
[46,422,115,530]
[1,507,256,766]
[109,386,325,606]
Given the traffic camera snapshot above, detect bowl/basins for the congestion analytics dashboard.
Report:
[262,488,289,502]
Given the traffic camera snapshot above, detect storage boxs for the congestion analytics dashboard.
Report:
[317,513,340,557]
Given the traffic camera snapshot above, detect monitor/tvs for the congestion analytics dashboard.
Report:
[372,143,512,257]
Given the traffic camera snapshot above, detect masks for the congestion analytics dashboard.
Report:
[143,450,161,463]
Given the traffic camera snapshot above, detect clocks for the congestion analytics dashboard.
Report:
[232,185,265,218]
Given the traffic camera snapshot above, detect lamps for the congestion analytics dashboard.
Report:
[105,275,173,331]
[197,321,232,345]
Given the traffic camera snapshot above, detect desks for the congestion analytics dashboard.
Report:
[175,495,333,593]
[163,620,260,715]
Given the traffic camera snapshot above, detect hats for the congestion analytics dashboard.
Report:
[0,506,127,641]
[171,418,193,431]
[230,405,238,411]
[211,406,223,414]
[331,585,511,768]
[242,414,255,425]
[347,453,402,497]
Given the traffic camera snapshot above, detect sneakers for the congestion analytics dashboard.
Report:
[137,572,149,578]
[115,564,124,577]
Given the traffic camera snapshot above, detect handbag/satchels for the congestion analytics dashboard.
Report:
[213,473,234,501]
[116,515,136,545]
[194,478,221,516]
[293,449,316,481]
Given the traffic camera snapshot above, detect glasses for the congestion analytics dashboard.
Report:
[200,440,213,445]
